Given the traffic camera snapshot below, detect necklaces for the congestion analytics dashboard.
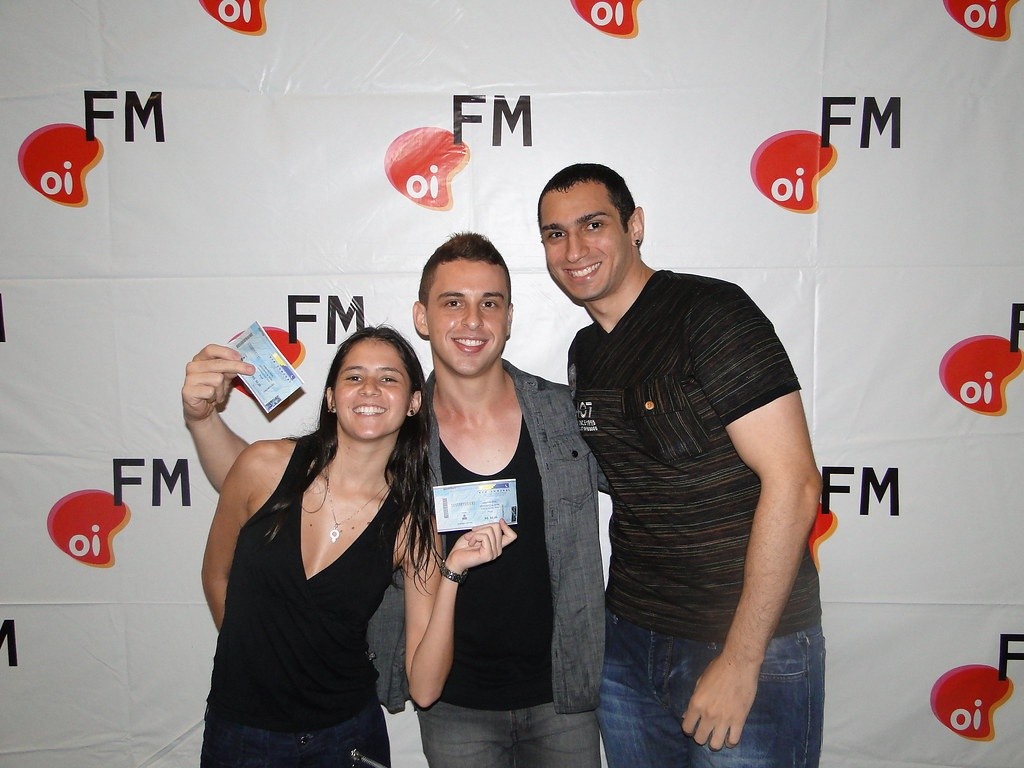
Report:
[326,467,386,544]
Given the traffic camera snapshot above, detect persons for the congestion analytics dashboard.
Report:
[200,327,517,768]
[537,163,826,768]
[182,233,610,768]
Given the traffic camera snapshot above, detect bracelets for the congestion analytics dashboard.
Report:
[440,559,468,584]
